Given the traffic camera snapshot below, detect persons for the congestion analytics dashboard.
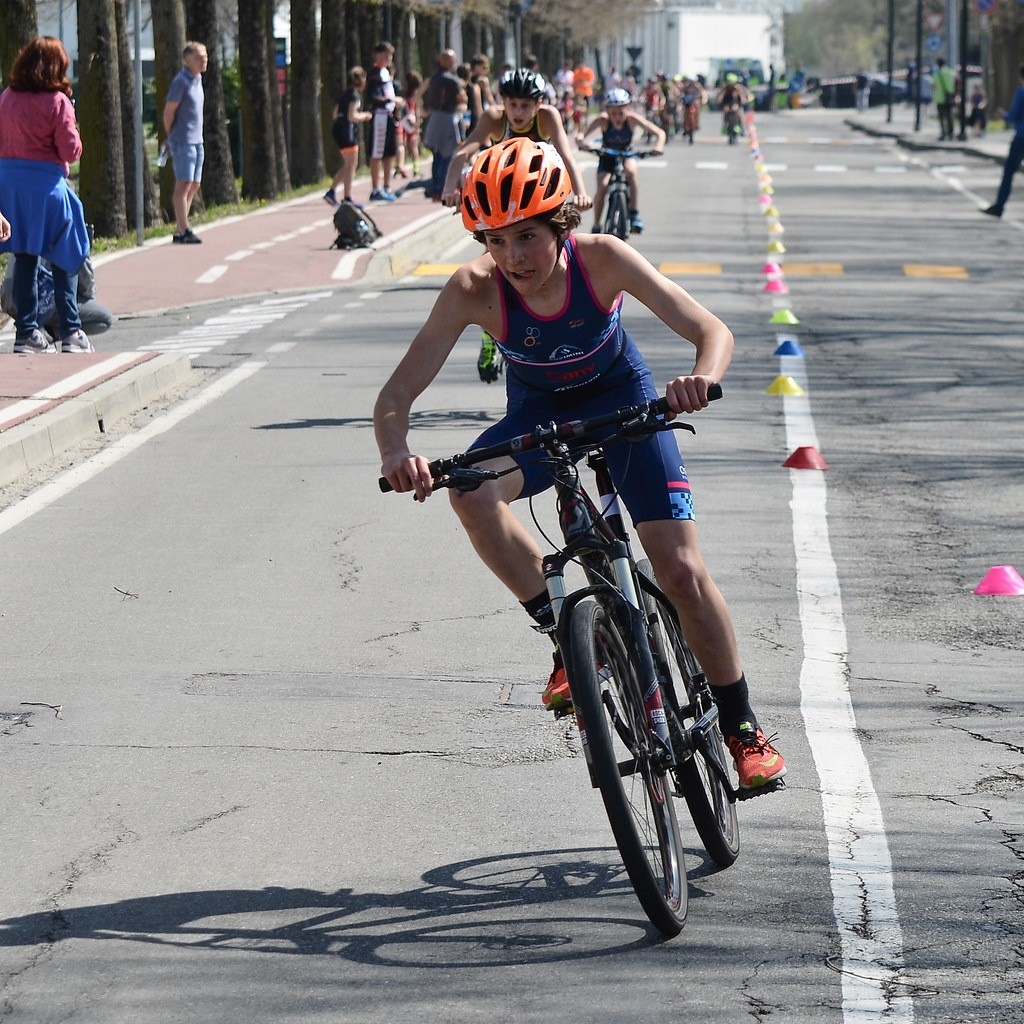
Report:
[768,65,777,111]
[576,88,667,234]
[443,67,592,212]
[163,41,208,244]
[979,66,1024,217]
[935,58,954,141]
[0,252,113,344]
[715,73,746,135]
[524,53,596,134]
[968,83,987,130]
[324,66,372,210]
[0,36,96,356]
[374,137,786,789]
[605,66,711,132]
[363,41,497,203]
[852,72,870,111]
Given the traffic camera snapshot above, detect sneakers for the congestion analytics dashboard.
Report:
[542,643,611,703]
[476,332,494,378]
[14,328,57,354]
[727,720,787,789]
[61,329,95,354]
[172,227,201,244]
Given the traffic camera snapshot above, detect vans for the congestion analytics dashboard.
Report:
[709,57,770,111]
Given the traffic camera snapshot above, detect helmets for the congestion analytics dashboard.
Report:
[460,137,572,230]
[604,87,631,105]
[498,67,547,101]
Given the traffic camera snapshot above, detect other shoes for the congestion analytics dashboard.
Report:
[980,203,1002,217]
[323,190,338,206]
[369,190,396,201]
[630,214,643,231]
[397,166,406,177]
[345,196,364,209]
[413,167,420,175]
[593,224,601,234]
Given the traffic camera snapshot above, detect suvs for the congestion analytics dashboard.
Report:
[822,74,909,109]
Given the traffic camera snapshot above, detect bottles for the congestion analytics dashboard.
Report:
[647,633,661,677]
[157,146,168,167]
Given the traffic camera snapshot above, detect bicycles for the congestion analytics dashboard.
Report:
[379,385,786,936]
[720,101,746,147]
[581,145,655,242]
[638,96,700,148]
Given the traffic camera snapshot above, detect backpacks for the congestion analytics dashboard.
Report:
[333,200,382,243]
